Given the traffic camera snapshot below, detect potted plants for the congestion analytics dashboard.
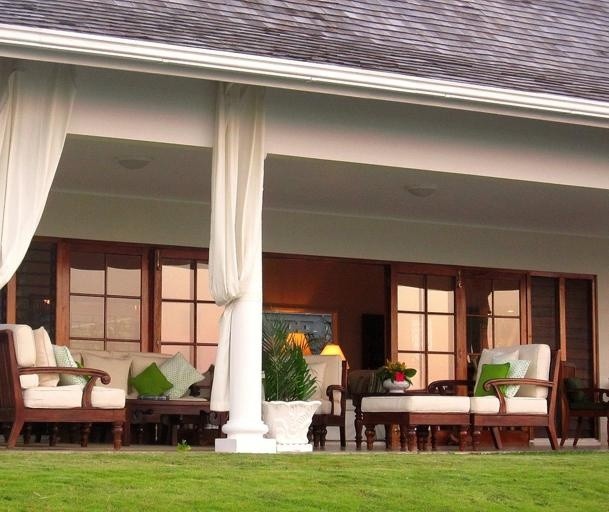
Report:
[261,314,325,453]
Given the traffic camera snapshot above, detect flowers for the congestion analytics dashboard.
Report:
[371,357,418,379]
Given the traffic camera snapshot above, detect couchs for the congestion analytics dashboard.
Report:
[0,322,208,451]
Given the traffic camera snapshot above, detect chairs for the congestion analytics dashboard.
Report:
[430,342,559,456]
[553,358,609,450]
[306,352,349,452]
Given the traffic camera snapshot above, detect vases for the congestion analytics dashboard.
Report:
[381,380,411,394]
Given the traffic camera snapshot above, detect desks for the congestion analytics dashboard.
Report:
[124,398,231,448]
[346,387,464,453]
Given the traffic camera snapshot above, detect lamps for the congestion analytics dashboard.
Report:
[113,156,151,174]
[278,332,312,355]
[319,343,347,362]
[406,183,439,200]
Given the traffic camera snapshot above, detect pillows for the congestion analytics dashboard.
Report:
[31,324,207,396]
[474,348,531,398]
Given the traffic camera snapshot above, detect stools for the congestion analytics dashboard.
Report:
[357,394,471,454]
